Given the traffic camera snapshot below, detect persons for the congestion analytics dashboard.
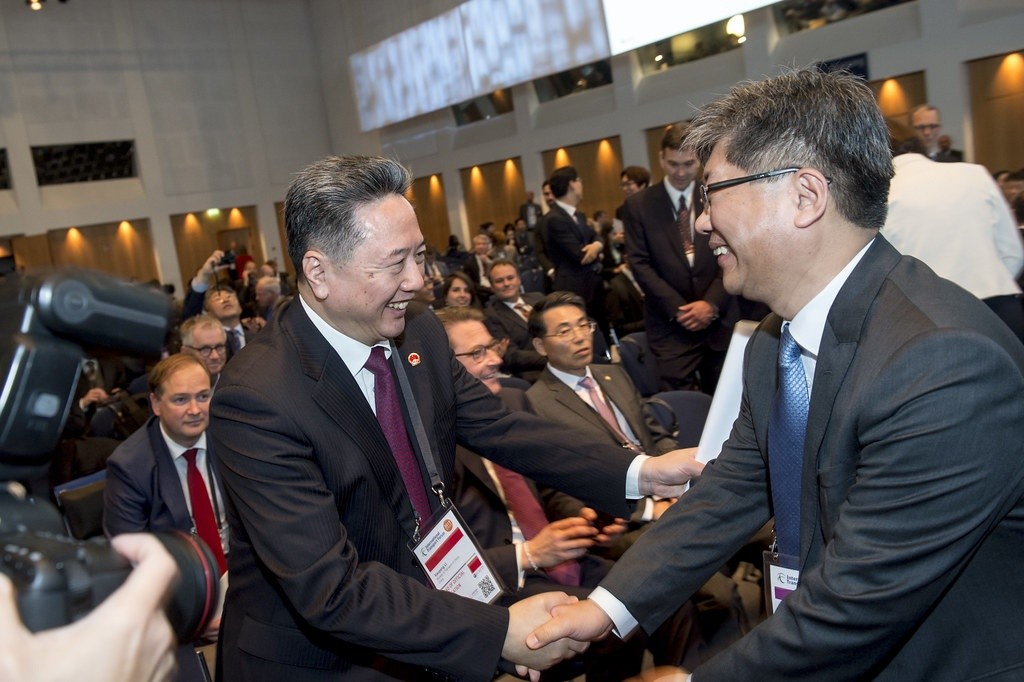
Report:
[0,67,1024,682]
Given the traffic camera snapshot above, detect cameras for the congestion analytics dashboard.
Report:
[218,254,236,266]
[0,262,220,647]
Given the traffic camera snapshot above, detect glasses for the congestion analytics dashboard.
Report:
[914,124,941,131]
[456,338,503,363]
[208,293,238,309]
[698,166,832,214]
[186,344,227,358]
[620,181,636,186]
[543,320,597,338]
[422,277,434,287]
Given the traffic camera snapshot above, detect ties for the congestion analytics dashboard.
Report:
[580,376,642,458]
[364,348,434,538]
[768,323,810,572]
[492,462,582,589]
[483,262,487,269]
[229,328,241,354]
[182,449,226,578]
[574,211,590,244]
[87,361,98,387]
[676,195,694,255]
[514,304,530,318]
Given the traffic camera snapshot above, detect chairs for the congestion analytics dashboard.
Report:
[61,479,105,540]
